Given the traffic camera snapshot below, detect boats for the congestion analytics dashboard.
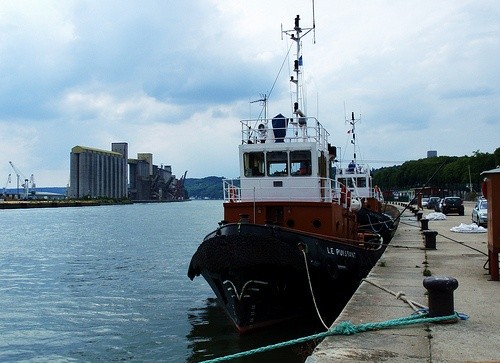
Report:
[186,0,403,334]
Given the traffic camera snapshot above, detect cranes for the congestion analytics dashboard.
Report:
[29,173,36,191]
[9,161,20,195]
[5,174,11,188]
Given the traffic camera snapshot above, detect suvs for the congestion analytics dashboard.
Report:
[441,197,464,216]
[427,197,440,209]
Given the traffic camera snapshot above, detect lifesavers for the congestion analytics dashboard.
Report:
[347,192,352,208]
[340,185,347,204]
[482,178,487,200]
[375,186,378,193]
[229,188,237,202]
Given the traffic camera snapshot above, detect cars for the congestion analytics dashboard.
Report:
[472,199,488,227]
[421,198,429,207]
[435,199,444,212]
